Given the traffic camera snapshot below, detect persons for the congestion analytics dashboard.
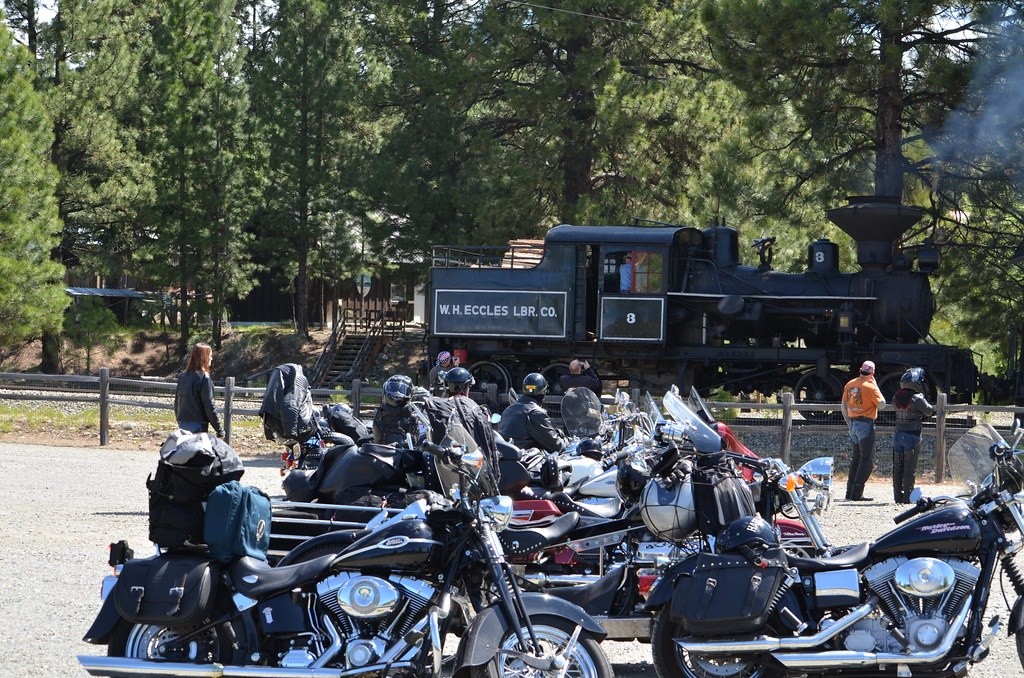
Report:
[498,373,569,454]
[443,368,482,442]
[173,343,225,440]
[371,374,428,444]
[429,351,451,393]
[560,360,599,437]
[841,361,886,500]
[65,302,73,312]
[621,253,646,291]
[891,367,933,503]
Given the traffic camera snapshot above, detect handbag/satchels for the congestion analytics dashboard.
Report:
[145,456,272,561]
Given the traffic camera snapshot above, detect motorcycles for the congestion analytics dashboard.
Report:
[77,384,835,678]
[643,414,1024,678]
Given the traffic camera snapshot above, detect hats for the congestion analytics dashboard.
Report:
[863,361,876,373]
[625,251,632,257]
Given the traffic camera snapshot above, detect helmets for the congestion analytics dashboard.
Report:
[332,402,352,417]
[900,368,928,393]
[382,375,413,407]
[444,367,476,386]
[638,468,698,541]
[522,373,548,394]
[576,438,604,456]
[716,516,780,555]
[615,460,654,510]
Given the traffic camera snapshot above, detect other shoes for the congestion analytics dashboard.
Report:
[850,496,873,501]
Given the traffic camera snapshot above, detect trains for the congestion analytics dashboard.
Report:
[423,195,983,420]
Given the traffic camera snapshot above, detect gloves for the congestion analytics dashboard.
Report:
[655,440,691,485]
